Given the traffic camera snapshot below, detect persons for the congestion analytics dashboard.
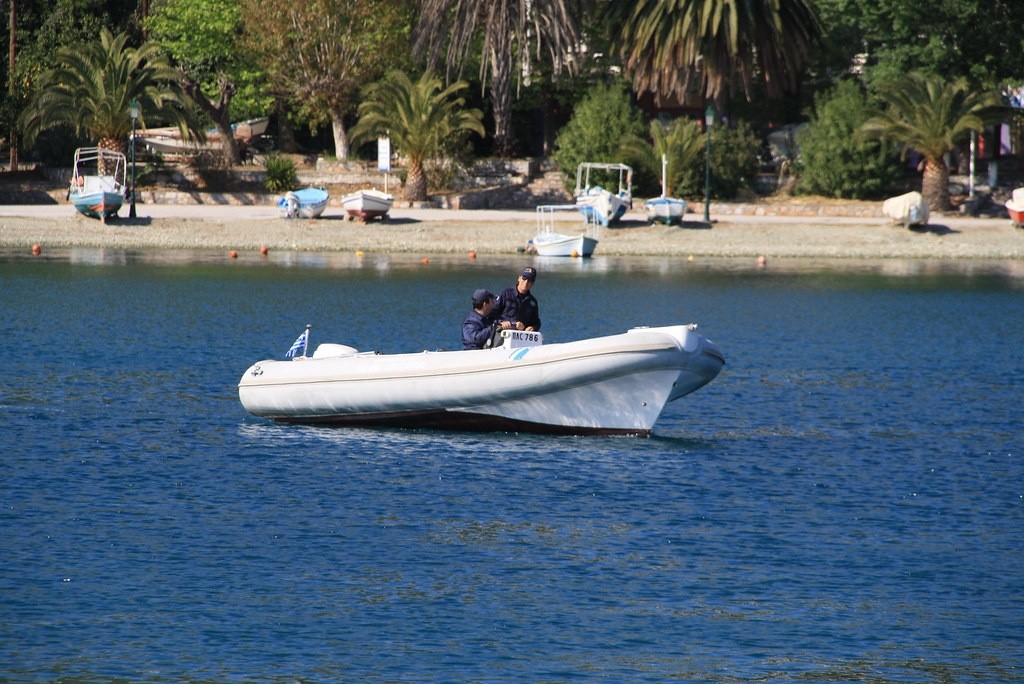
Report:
[461,267,542,350]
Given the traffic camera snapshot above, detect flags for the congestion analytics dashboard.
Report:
[284,329,306,357]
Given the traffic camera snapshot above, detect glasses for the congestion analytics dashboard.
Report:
[522,276,534,283]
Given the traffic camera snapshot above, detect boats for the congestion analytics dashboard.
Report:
[341,187,395,222]
[643,152,686,227]
[277,186,331,220]
[128,118,270,153]
[237,323,727,438]
[884,191,930,228]
[1006,188,1023,225]
[69,147,129,223]
[572,162,633,228]
[532,204,600,257]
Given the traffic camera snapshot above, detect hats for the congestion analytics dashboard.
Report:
[520,267,537,281]
[472,289,499,305]
